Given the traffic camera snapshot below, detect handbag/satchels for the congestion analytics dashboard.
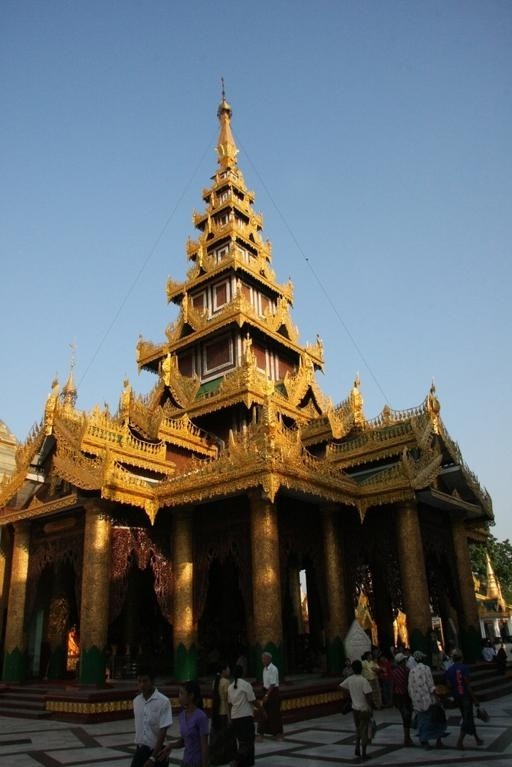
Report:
[368,718,377,738]
[429,705,448,729]
[463,678,479,703]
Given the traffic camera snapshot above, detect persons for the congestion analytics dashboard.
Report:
[130,652,284,766]
[336,628,512,760]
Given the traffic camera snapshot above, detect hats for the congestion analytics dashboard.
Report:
[395,653,407,662]
[452,648,462,657]
[413,651,426,657]
